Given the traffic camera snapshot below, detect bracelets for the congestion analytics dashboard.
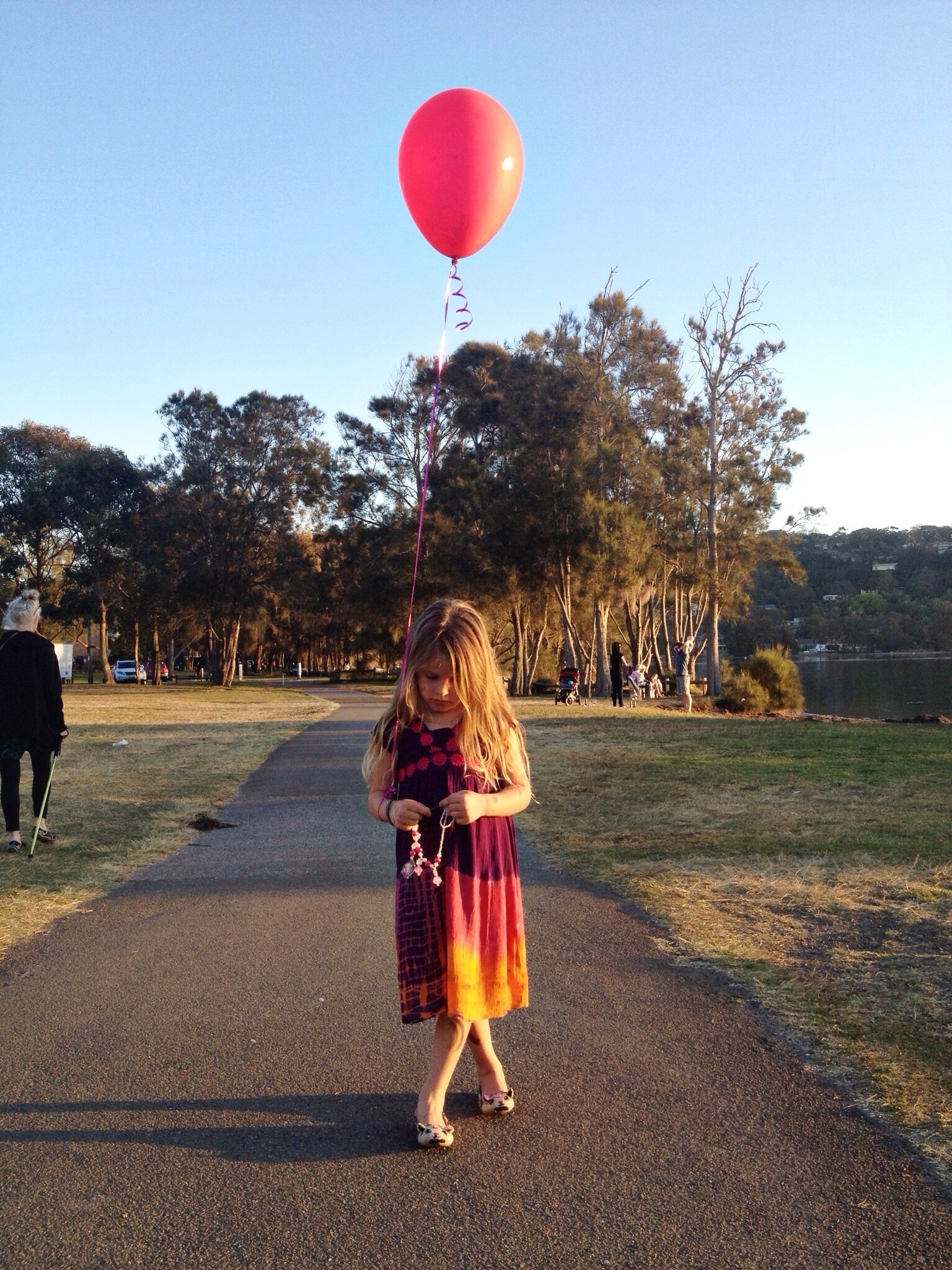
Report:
[378,785,396,823]
[386,798,397,826]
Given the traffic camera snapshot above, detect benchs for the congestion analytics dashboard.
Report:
[636,680,652,700]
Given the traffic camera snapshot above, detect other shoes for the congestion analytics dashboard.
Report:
[414,1108,454,1147]
[478,1084,515,1115]
[31,827,55,841]
[7,840,26,853]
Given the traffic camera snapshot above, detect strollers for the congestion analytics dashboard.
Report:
[555,667,582,705]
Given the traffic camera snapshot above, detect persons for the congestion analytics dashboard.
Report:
[610,641,629,709]
[0,589,69,851]
[634,664,666,698]
[195,662,200,677]
[673,635,695,714]
[362,598,532,1146]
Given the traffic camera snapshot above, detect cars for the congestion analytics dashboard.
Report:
[111,660,170,685]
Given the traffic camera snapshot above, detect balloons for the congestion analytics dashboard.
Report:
[397,88,524,264]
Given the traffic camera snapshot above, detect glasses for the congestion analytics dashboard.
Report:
[675,647,679,650]
[619,646,621,648]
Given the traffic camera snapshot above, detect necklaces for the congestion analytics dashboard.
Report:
[427,714,459,725]
[401,804,456,886]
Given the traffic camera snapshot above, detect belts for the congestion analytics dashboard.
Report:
[676,675,685,677]
